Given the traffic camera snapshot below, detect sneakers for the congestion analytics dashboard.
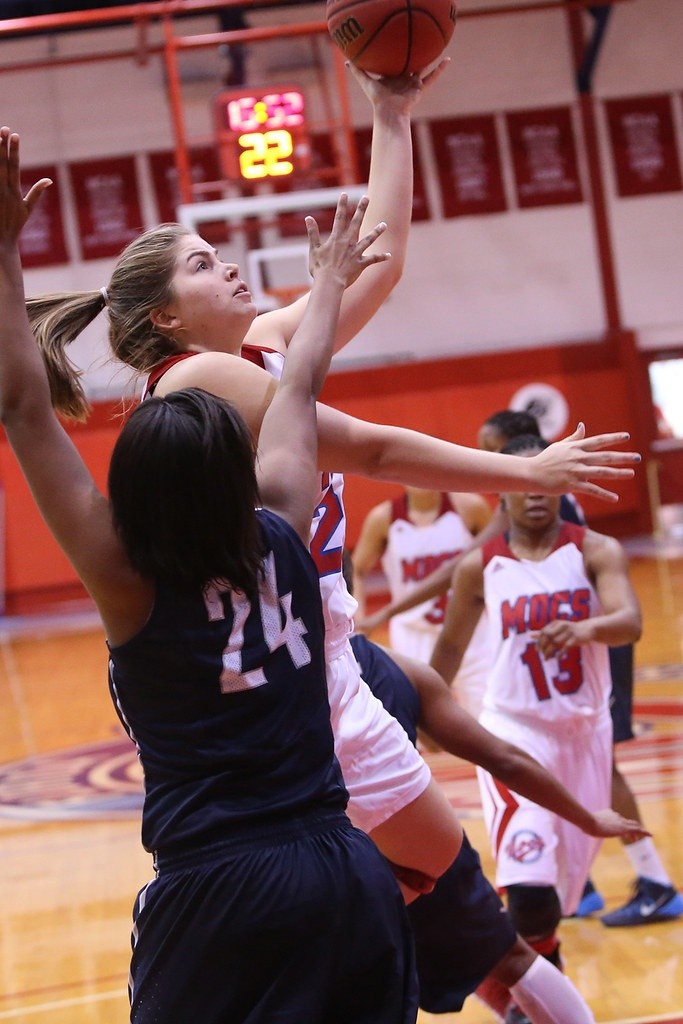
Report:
[571,881,603,917]
[601,876,683,926]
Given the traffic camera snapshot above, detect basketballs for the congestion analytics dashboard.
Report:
[325,0,457,76]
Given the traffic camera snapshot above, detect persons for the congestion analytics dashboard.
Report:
[1,127,420,1024]
[347,633,653,838]
[350,483,491,756]
[478,413,682,927]
[426,436,644,1024]
[23,57,642,1023]
